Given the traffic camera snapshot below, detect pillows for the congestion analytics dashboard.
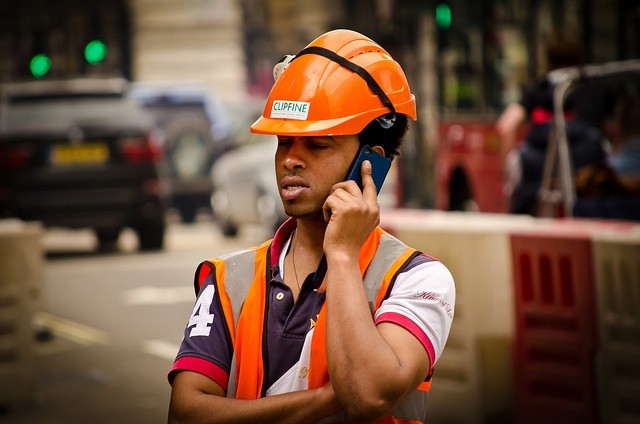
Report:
[250,29,417,137]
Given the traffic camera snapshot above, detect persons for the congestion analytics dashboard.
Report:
[168,28,457,423]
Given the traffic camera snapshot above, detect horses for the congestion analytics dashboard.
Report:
[343,142,392,198]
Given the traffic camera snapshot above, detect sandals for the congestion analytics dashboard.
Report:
[432,0,640,220]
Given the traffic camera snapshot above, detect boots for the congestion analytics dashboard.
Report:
[0,76,163,250]
[134,85,235,223]
